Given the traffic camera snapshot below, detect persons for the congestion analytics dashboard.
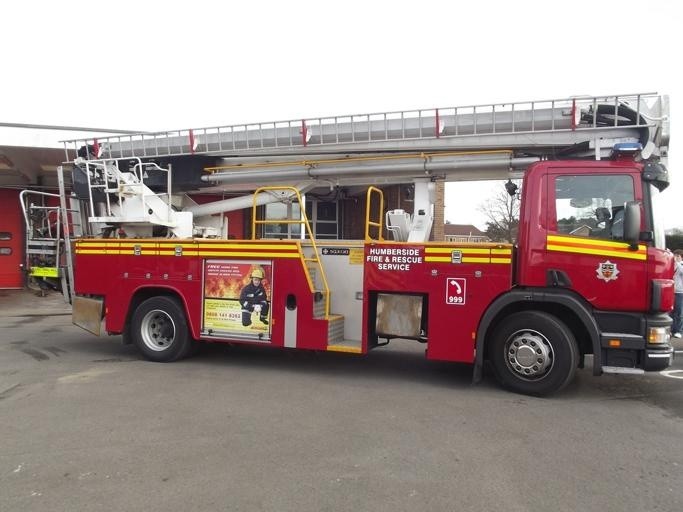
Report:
[673,249,683,338]
[237,271,269,327]
[590,206,612,238]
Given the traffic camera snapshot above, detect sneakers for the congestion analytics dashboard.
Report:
[674,332,681,339]
[260,317,268,324]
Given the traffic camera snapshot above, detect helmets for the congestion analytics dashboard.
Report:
[250,270,263,279]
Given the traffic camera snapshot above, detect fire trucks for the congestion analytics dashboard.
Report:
[57,90,675,396]
